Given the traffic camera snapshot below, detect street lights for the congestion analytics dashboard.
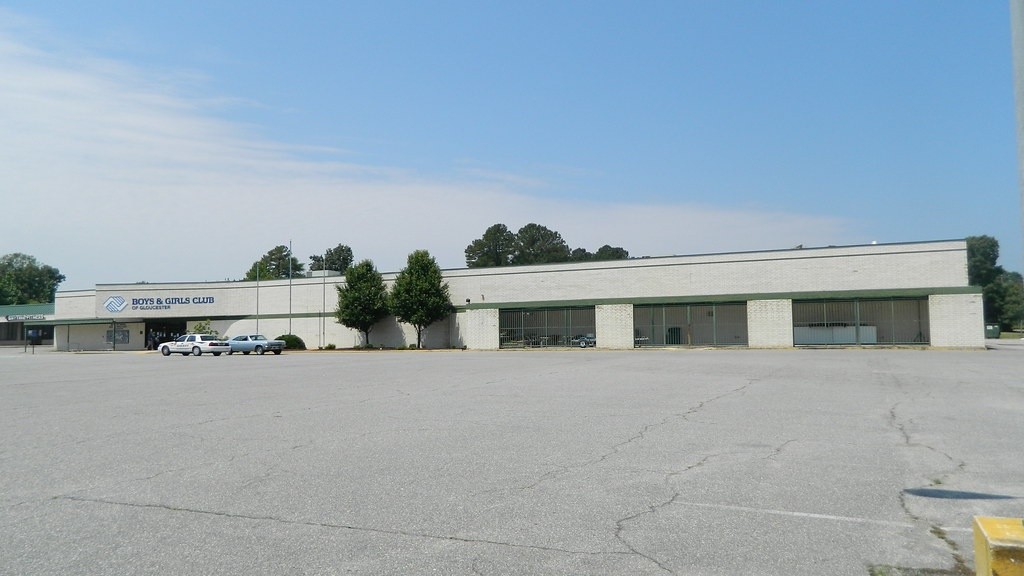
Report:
[522,311,531,347]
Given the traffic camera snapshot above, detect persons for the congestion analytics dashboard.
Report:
[146,329,155,350]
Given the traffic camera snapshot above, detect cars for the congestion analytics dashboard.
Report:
[571,335,597,347]
[158,333,231,356]
[224,334,287,355]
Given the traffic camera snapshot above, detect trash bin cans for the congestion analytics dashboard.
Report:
[984,323,1001,338]
